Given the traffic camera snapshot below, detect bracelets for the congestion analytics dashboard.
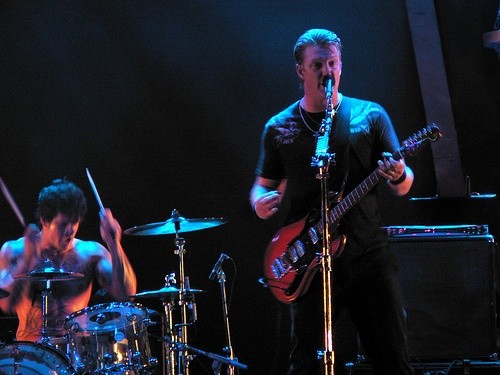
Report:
[389,170,407,187]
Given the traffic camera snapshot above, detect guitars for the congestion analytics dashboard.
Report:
[262,119,442,303]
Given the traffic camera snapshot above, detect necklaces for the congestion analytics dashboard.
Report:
[299,91,343,143]
[39,241,67,268]
[302,97,341,127]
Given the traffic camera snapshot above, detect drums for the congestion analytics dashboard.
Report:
[63,302,148,375]
[0,340,78,374]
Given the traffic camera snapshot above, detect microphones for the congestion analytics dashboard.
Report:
[209,253,228,280]
[322,75,335,98]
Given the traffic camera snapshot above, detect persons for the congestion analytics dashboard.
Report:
[0,177,137,346]
[250,29,415,375]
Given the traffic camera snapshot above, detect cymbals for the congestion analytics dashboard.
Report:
[124,208,230,237]
[11,257,86,281]
[133,273,203,302]
